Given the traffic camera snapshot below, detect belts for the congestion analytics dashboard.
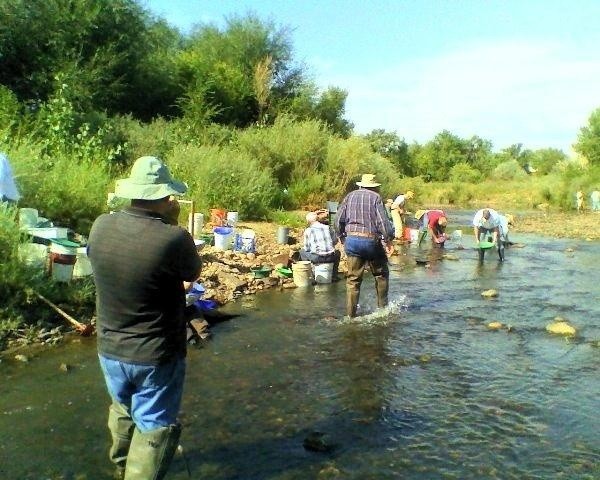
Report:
[347,231,377,240]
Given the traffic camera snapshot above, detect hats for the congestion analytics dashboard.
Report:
[114,155,189,202]
[407,190,414,199]
[356,174,382,188]
[415,209,426,220]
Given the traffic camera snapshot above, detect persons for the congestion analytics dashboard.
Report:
[575,188,584,212]
[86,156,200,480]
[590,187,600,211]
[298,173,513,317]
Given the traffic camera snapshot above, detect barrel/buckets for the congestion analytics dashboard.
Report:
[315,263,334,283]
[291,261,314,287]
[277,227,289,244]
[73,248,93,278]
[50,242,77,278]
[227,212,238,227]
[210,208,225,226]
[189,213,204,236]
[214,227,234,250]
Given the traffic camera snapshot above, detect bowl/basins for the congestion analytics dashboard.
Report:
[471,241,496,252]
[433,233,447,244]
[250,267,274,279]
[193,238,206,252]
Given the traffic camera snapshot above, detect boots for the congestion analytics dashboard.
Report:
[108,401,181,479]
[498,248,505,262]
[478,249,486,261]
[332,260,342,281]
[344,255,367,318]
[417,232,426,247]
[368,257,390,311]
